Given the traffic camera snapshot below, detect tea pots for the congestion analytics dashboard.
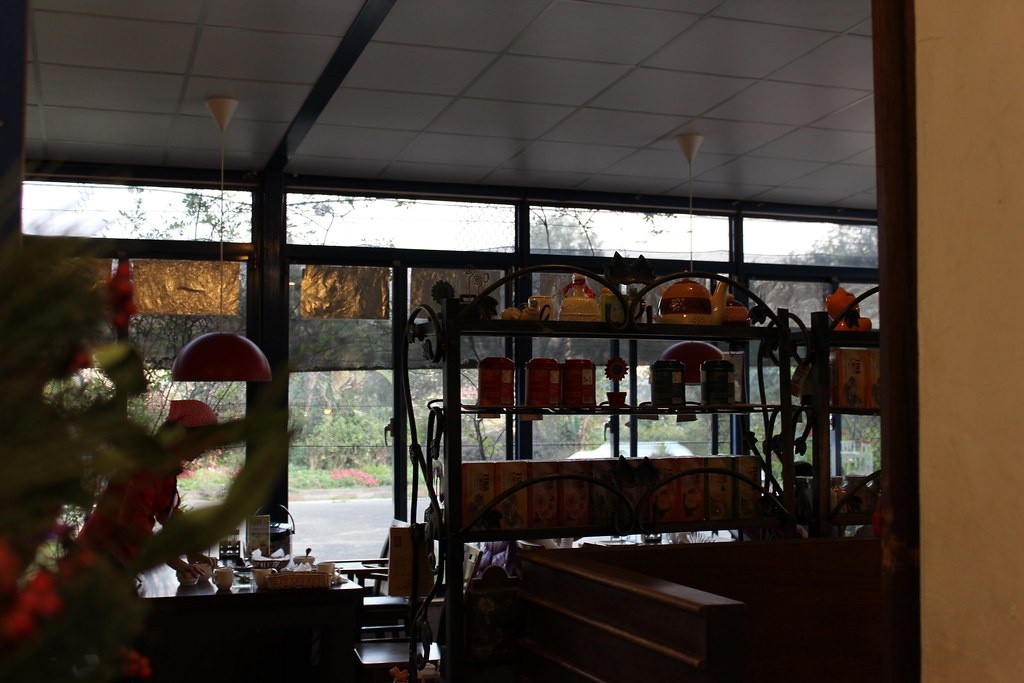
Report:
[653,271,728,325]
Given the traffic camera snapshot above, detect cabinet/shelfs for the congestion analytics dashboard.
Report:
[393,284,882,681]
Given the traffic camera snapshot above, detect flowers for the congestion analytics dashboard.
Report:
[0,239,297,683]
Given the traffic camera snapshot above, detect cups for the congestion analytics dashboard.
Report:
[211,568,233,589]
[293,556,314,565]
[318,563,340,581]
[193,563,213,582]
[176,571,199,585]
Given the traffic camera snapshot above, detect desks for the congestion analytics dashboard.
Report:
[138,553,363,683]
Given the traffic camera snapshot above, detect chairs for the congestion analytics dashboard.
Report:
[325,518,418,596]
[363,546,482,665]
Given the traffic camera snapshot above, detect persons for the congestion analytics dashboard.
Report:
[672,531,738,544]
[744,463,840,540]
[70,400,218,578]
[474,536,574,579]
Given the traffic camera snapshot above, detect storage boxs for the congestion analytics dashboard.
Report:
[458,457,762,528]
[835,348,880,408]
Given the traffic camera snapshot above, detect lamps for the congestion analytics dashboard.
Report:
[660,135,713,314]
[171,97,271,381]
